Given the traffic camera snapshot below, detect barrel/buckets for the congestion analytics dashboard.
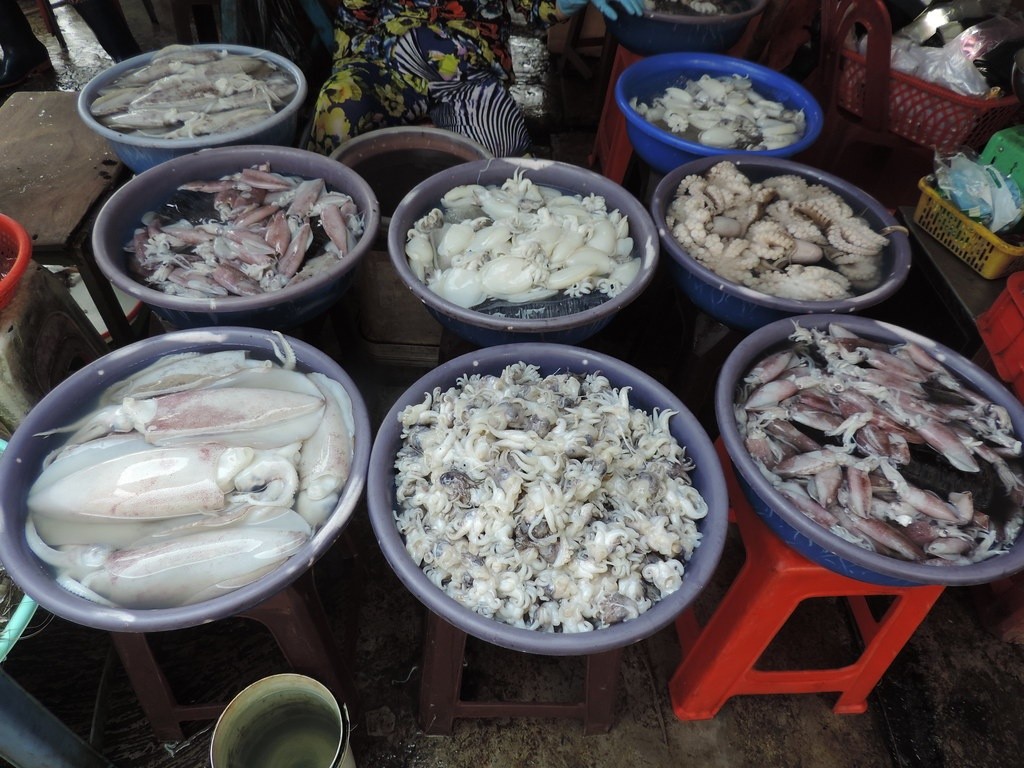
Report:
[210,674,357,768]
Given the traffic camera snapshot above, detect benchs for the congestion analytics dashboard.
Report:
[296,249,476,370]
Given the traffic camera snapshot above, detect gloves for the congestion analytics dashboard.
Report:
[557,0,646,21]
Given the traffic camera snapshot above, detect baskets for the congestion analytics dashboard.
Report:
[832,48,1024,158]
[914,174,1024,280]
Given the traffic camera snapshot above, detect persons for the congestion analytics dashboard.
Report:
[307,0,645,159]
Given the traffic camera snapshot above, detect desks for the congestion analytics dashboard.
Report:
[896,205,1008,374]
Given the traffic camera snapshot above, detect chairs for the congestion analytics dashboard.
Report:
[805,0,936,219]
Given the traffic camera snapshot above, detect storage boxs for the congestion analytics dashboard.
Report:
[974,272,1023,405]
[976,126,1024,199]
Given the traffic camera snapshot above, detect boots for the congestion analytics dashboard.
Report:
[0,1,53,92]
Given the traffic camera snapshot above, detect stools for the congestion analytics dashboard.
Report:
[422,610,624,737]
[117,540,354,742]
[584,0,812,186]
[667,439,948,720]
[1,92,149,347]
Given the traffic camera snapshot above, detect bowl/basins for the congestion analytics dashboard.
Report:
[388,158,659,345]
[615,52,823,174]
[0,324,370,632]
[93,146,381,336]
[604,0,767,56]
[366,346,728,658]
[714,314,1024,589]
[78,44,308,174]
[652,155,910,331]
